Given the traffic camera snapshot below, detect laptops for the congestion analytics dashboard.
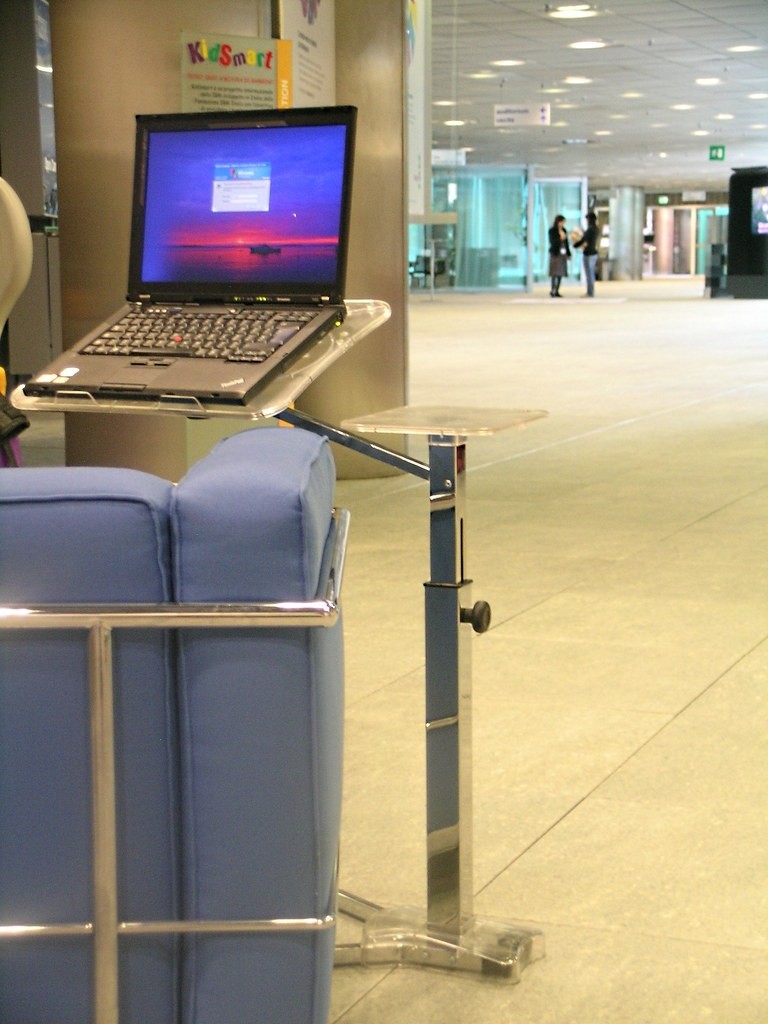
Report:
[22,105,358,406]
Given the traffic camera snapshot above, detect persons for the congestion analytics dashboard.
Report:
[572,211,600,298]
[547,214,570,297]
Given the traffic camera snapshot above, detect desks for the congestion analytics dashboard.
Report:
[7,293,548,983]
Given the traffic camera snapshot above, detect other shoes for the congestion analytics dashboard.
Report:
[550,291,562,297]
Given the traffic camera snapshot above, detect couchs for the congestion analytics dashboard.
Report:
[0,426,342,1024]
